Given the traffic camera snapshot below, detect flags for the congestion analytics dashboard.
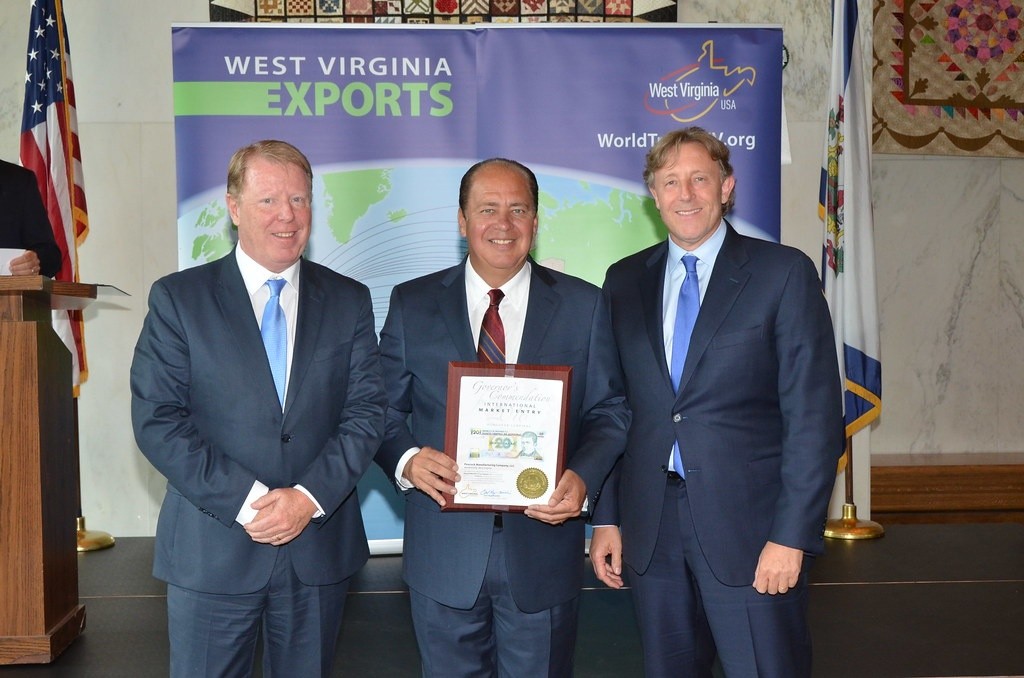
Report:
[18,0,91,398]
[814,0,877,484]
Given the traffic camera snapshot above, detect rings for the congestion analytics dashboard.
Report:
[31,267,35,275]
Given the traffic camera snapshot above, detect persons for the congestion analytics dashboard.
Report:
[126,140,390,678]
[364,160,635,676]
[1,160,62,281]
[583,126,844,678]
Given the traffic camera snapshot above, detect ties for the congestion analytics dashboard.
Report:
[476,290,506,367]
[671,256,699,480]
[260,279,287,412]
